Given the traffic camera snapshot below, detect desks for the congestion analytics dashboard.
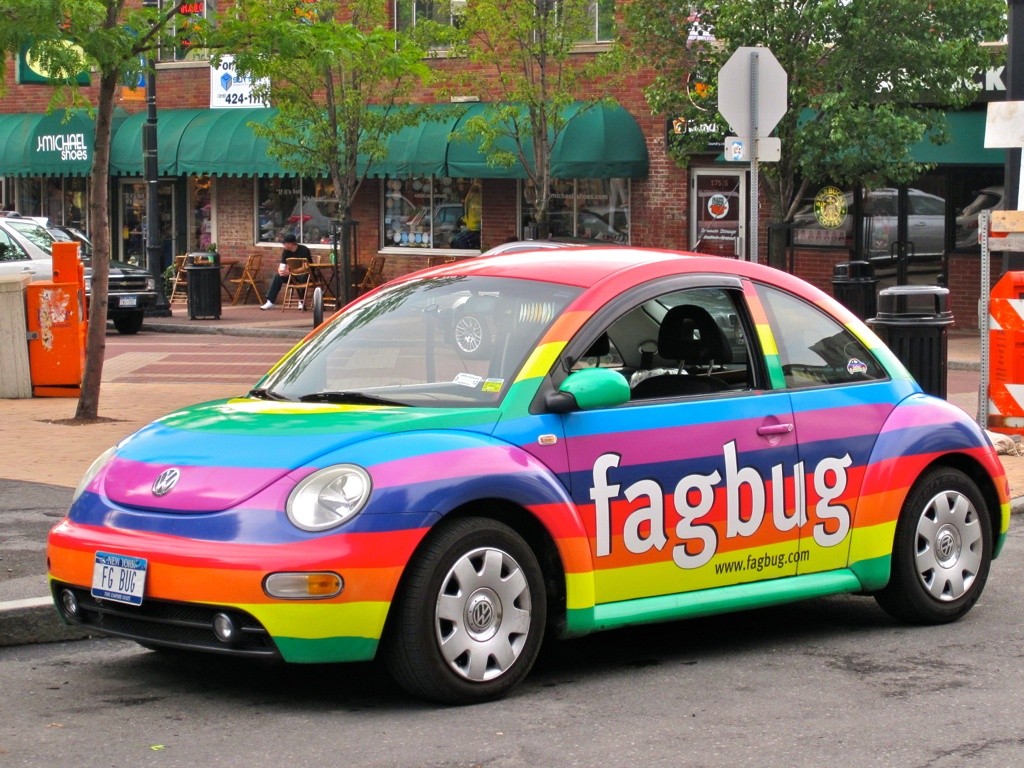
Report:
[309,263,363,297]
[194,261,240,301]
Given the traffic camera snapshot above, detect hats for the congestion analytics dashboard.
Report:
[281,235,296,242]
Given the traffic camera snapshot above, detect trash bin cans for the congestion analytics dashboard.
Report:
[0,275,33,398]
[184,264,225,319]
[864,285,954,400]
[27,242,88,397]
[833,260,880,329]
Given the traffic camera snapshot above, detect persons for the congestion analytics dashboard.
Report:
[259,234,312,310]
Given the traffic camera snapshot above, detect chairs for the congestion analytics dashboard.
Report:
[352,257,387,296]
[569,332,634,378]
[230,254,265,306]
[632,305,733,399]
[170,253,201,304]
[282,257,325,311]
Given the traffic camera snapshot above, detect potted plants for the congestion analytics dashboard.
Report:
[208,243,220,266]
[330,249,339,264]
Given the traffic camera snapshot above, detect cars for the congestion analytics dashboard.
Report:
[0,209,159,335]
[789,187,982,255]
[44,236,1012,708]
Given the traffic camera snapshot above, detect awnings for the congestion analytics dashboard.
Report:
[715,107,1008,166]
[0,107,130,178]
[106,97,650,179]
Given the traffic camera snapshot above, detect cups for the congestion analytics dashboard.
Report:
[280,264,286,271]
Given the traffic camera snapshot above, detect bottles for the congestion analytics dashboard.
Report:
[330,249,334,264]
[524,179,537,204]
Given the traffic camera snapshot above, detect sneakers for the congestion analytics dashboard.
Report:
[298,302,305,309]
[260,299,274,309]
